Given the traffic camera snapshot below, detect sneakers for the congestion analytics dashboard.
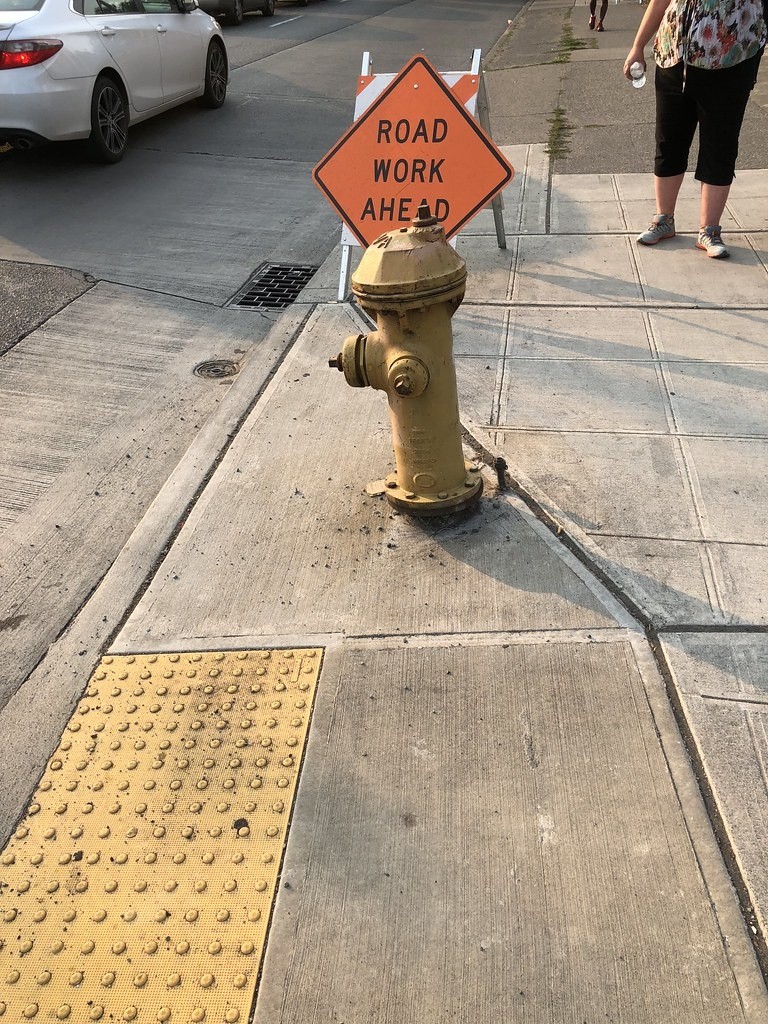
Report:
[636,214,675,245]
[695,224,728,258]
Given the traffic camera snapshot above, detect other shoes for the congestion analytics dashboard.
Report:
[597,22,604,32]
[588,15,596,29]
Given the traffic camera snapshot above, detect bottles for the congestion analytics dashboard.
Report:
[629,62,646,88]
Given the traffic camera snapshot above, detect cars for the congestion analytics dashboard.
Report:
[1,0,276,166]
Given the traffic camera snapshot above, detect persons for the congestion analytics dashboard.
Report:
[623,0,768,258]
[588,0,608,32]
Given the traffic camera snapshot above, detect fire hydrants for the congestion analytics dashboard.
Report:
[327,206,485,521]
